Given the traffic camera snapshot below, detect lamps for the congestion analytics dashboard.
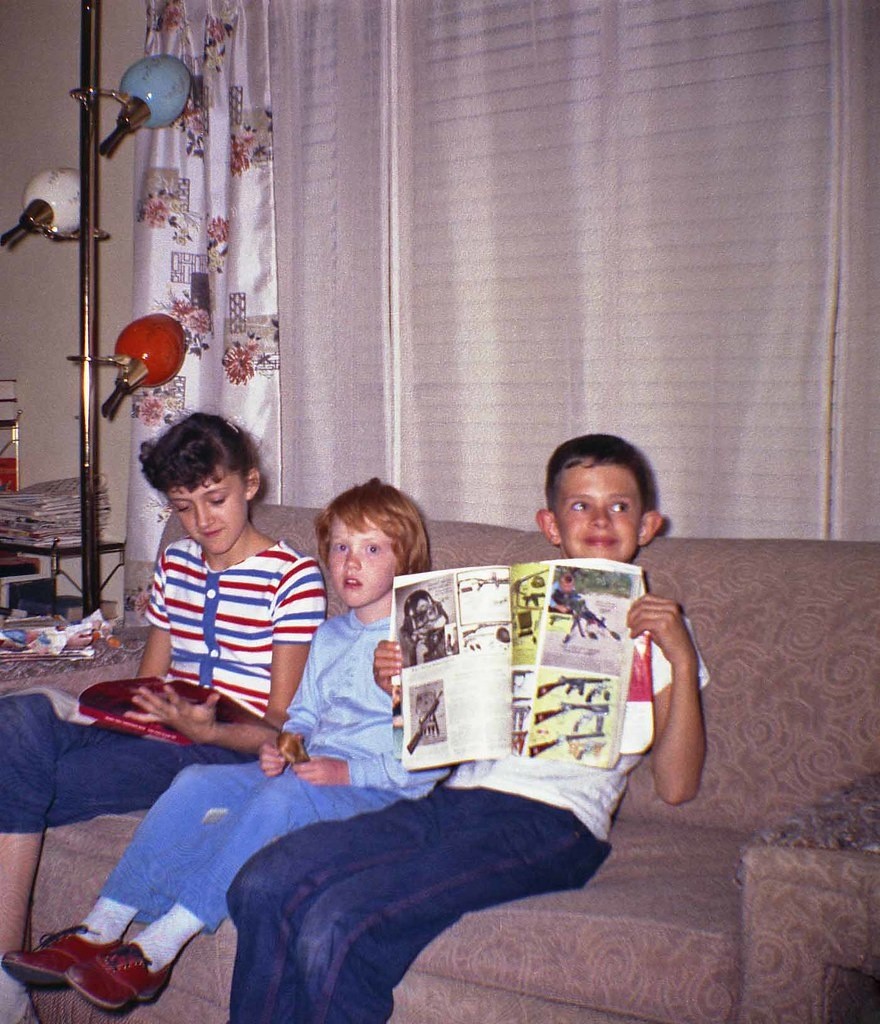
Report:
[0,168,81,246]
[69,54,191,158]
[66,313,187,419]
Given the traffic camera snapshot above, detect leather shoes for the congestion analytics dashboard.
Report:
[2,925,121,986]
[65,943,172,1009]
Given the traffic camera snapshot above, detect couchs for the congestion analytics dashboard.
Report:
[0,501,880,1024]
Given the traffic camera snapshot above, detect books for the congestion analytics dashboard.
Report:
[0,614,107,661]
[0,474,110,546]
[392,559,657,772]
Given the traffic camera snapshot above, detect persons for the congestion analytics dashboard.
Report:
[225,434,711,1024]
[1,478,449,1010]
[1,412,327,1024]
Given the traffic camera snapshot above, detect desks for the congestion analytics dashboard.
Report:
[0,538,127,627]
